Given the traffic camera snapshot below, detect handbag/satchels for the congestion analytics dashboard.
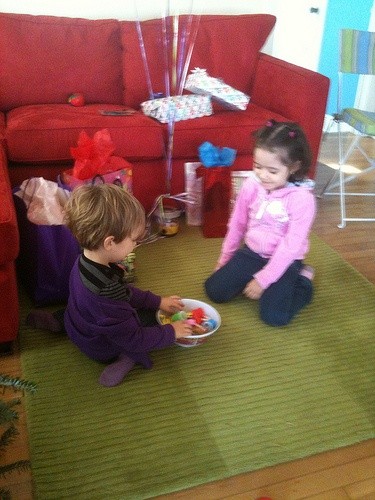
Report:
[196,162,232,238]
[12,176,80,309]
[59,165,134,195]
[141,93,212,123]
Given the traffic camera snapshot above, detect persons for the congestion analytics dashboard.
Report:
[25,183,193,388]
[204,117,317,327]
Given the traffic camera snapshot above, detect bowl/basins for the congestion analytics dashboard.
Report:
[156,299,222,348]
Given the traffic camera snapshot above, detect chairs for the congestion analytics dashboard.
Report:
[316,27,375,230]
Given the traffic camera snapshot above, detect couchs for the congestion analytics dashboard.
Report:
[0,14,330,343]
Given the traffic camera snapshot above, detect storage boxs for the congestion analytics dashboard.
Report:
[141,94,213,123]
[60,162,134,195]
[184,71,252,112]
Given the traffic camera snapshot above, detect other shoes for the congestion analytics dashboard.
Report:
[300,264,316,280]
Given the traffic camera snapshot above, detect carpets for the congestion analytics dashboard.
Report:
[22,213,375,500]
